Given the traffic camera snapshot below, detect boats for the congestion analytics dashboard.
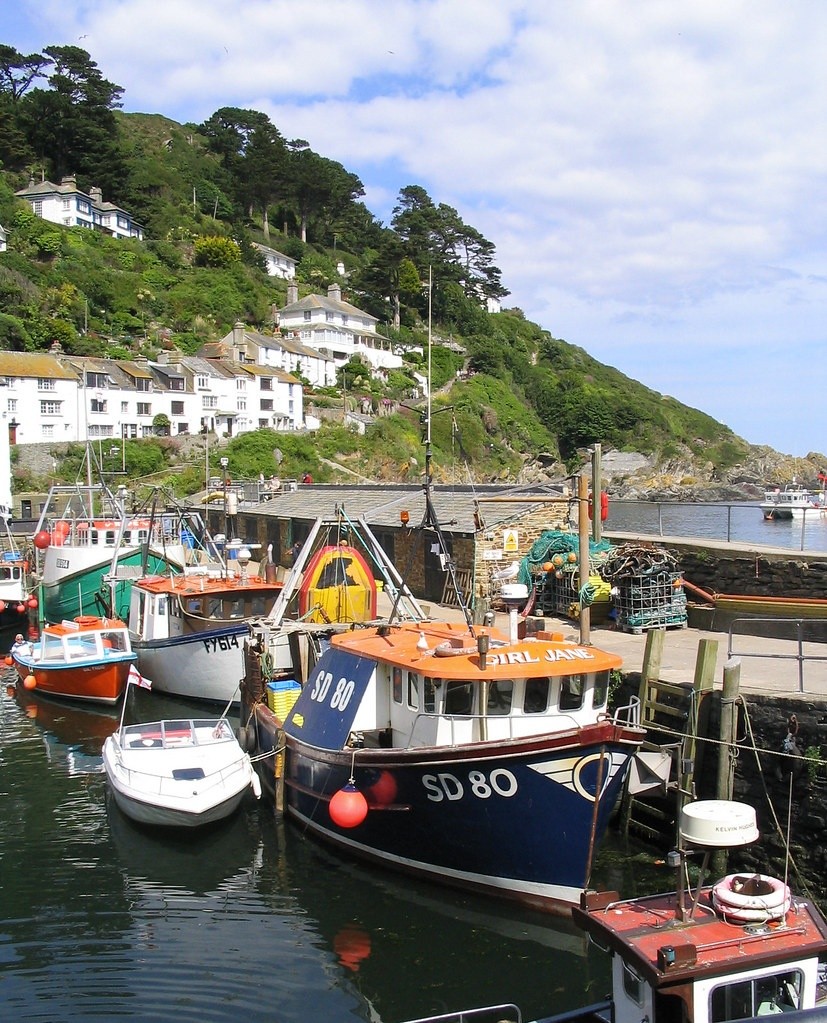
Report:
[28,438,187,628]
[103,717,260,831]
[402,763,827,1023]
[759,476,826,522]
[101,486,384,716]
[237,396,694,928]
[0,505,34,629]
[8,581,139,709]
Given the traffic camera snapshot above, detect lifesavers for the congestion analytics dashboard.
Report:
[74,615,98,626]
[710,872,791,920]
[137,576,166,584]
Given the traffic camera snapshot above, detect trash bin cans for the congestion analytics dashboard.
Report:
[181,530,194,548]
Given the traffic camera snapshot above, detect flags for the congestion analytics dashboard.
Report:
[129,664,153,691]
